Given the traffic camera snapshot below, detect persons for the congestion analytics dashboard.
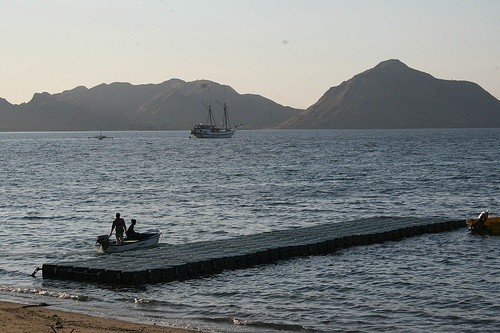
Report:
[126,219,136,237]
[478,210,489,222]
[111,213,127,246]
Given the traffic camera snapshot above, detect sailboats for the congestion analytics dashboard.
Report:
[190,84,236,138]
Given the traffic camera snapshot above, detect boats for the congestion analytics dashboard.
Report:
[465,210,500,237]
[94,228,163,253]
